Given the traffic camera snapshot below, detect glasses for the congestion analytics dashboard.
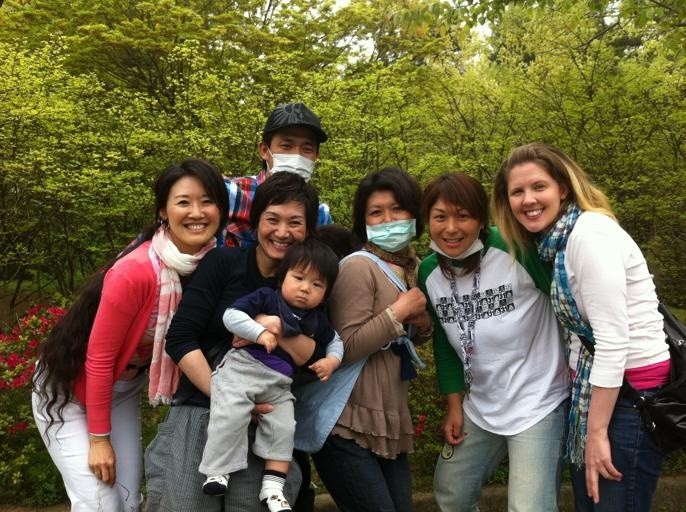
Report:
[97,479,134,512]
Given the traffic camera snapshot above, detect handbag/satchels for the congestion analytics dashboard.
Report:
[639,302,684,455]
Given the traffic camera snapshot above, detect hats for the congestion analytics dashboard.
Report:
[262,103,329,143]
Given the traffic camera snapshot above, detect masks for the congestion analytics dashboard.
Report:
[365,217,417,254]
[264,143,316,184]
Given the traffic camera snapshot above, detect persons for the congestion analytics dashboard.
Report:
[310,166,434,512]
[141,172,344,511]
[417,170,573,511]
[196,238,363,511]
[30,159,227,512]
[119,101,334,512]
[488,141,671,512]
